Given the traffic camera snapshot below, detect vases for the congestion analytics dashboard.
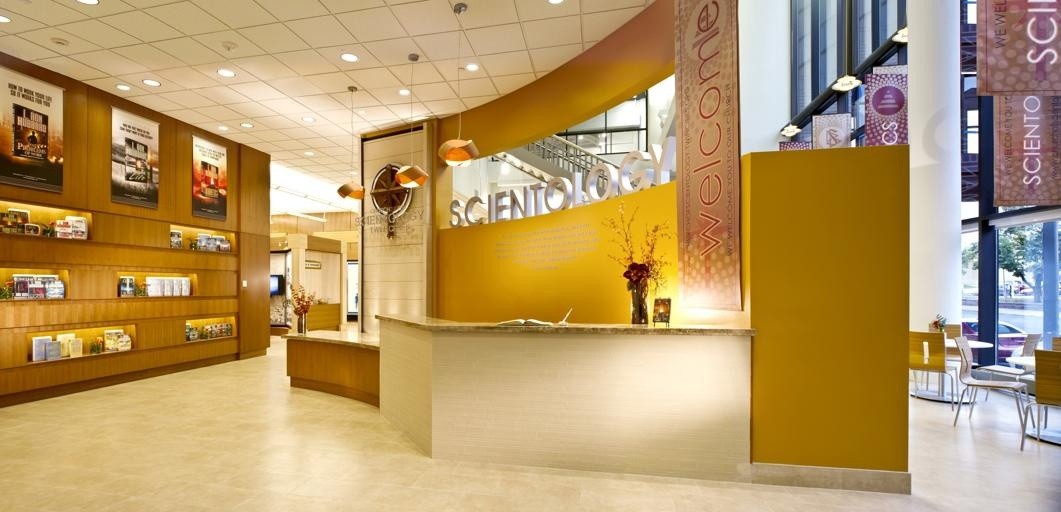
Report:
[630,282,649,324]
[298,312,306,334]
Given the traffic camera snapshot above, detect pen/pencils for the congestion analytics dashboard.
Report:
[558,308,574,321]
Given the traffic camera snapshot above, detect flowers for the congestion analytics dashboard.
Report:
[281,281,317,316]
[600,198,674,297]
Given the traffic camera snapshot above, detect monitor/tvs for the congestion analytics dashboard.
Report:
[269,275,283,295]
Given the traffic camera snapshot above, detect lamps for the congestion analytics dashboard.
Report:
[337,1,481,200]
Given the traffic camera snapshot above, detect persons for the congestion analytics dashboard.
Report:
[28,130,38,143]
[121,277,134,297]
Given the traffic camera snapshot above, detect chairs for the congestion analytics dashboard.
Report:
[908,320,1061,452]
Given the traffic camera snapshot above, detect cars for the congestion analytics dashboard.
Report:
[962,319,1034,370]
[997,279,1061,297]
